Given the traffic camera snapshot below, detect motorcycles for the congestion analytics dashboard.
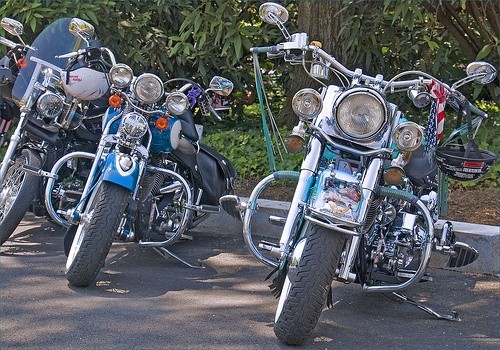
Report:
[55,18,239,286]
[0,15,118,245]
[219,0,497,346]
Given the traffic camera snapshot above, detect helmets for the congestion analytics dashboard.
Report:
[0,56,14,87]
[59,67,111,100]
[435,143,497,182]
